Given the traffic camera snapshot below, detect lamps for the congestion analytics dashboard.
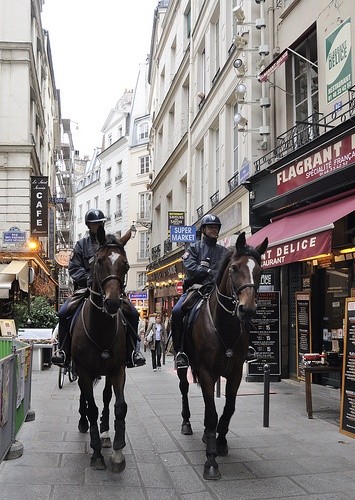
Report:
[178,273,185,280]
[233,6,256,27]
[232,35,258,52]
[234,85,260,104]
[169,280,174,287]
[233,113,259,133]
[233,59,257,78]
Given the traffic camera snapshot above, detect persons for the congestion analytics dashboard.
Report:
[136,316,146,352]
[48,209,146,367]
[171,214,257,366]
[146,315,167,372]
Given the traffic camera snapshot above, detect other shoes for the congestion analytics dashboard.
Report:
[244,351,258,360]
[158,367,162,371]
[50,353,69,368]
[177,357,187,366]
[127,354,145,368]
[153,369,157,372]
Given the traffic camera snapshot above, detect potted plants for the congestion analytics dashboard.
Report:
[11,296,59,362]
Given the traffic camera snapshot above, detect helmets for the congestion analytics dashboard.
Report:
[85,210,106,224]
[200,214,221,232]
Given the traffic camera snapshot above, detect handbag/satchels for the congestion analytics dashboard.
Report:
[147,330,154,342]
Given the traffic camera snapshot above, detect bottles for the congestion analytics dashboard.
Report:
[321,348,326,365]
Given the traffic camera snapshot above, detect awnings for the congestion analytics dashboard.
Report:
[257,20,318,96]
[244,193,355,269]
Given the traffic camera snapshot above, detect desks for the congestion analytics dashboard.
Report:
[302,366,342,419]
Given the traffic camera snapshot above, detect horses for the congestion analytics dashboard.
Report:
[171,231,269,480]
[71,224,128,474]
